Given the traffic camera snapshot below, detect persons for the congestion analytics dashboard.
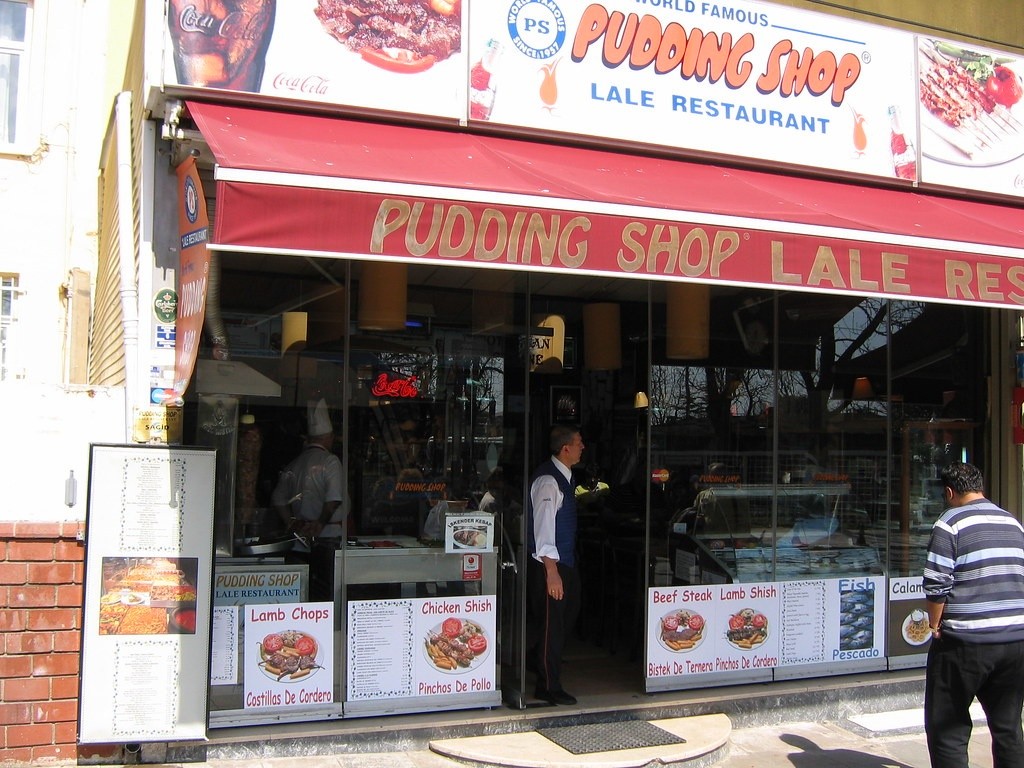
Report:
[942,419,985,476]
[254,407,303,508]
[525,424,585,705]
[370,467,523,550]
[923,462,1024,768]
[656,445,689,502]
[271,398,350,603]
[694,462,752,535]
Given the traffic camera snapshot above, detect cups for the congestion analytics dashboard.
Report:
[167,0,277,94]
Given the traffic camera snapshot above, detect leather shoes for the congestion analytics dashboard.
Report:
[535,687,577,705]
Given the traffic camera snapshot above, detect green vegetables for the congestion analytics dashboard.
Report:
[966,62,993,81]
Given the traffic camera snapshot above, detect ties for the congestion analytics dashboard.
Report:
[570,473,577,490]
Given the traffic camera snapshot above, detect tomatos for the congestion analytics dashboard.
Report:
[987,66,1023,105]
[263,634,283,652]
[753,614,765,627]
[295,638,313,654]
[442,617,462,636]
[688,615,703,629]
[729,616,744,629]
[468,636,486,653]
[665,617,678,630]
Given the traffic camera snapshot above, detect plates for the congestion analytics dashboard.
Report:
[920,61,1024,167]
[256,629,325,683]
[654,608,708,654]
[101,594,146,605]
[725,609,771,651]
[421,618,491,675]
[901,610,932,646]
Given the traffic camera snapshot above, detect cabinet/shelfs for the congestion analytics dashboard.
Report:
[669,484,882,586]
[770,414,981,581]
[354,397,450,534]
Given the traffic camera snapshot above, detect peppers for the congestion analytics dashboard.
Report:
[927,38,1017,64]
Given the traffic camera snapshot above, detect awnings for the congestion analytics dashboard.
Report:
[171,100,1023,310]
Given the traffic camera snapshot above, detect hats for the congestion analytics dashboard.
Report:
[306,398,333,436]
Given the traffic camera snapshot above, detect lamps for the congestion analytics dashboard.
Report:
[633,392,649,408]
[477,268,515,335]
[358,261,407,330]
[666,282,711,358]
[582,304,622,370]
[853,377,875,400]
[530,312,566,374]
[282,312,307,355]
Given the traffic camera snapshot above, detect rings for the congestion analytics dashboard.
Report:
[552,591,554,593]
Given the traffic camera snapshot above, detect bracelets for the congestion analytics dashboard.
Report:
[929,624,941,630]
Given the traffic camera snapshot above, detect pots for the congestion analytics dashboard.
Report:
[243,537,307,554]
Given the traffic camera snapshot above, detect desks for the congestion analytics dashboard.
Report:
[334,545,499,702]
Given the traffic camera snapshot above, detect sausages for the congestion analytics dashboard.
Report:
[732,633,762,648]
[428,645,457,669]
[665,634,702,651]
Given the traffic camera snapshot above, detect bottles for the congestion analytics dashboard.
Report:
[886,105,917,180]
[470,39,507,120]
